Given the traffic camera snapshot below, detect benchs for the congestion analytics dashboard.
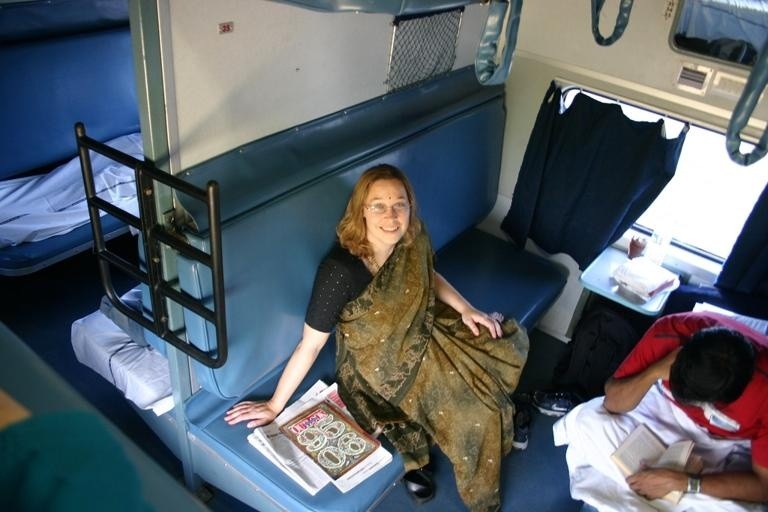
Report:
[173,64,567,512]
[0,29,144,276]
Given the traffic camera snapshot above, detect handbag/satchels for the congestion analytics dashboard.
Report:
[279,1,635,85]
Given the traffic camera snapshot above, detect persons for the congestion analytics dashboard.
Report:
[220,159,538,510]
[599,306,768,505]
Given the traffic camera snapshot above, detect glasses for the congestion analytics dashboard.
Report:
[363,201,412,215]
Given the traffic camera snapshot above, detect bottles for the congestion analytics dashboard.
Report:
[627,235,647,260]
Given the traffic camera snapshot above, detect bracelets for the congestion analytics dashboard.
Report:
[686,478,703,493]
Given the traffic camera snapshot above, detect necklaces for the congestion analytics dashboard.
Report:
[364,250,382,274]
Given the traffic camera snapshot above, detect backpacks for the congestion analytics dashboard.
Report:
[554,306,638,403]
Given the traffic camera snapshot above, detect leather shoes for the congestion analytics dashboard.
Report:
[417,452,438,477]
[402,463,435,504]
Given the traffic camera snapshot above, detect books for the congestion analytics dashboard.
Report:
[323,446,393,493]
[279,400,382,480]
[612,428,708,504]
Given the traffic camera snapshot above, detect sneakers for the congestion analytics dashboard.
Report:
[529,389,583,418]
[510,393,532,450]
